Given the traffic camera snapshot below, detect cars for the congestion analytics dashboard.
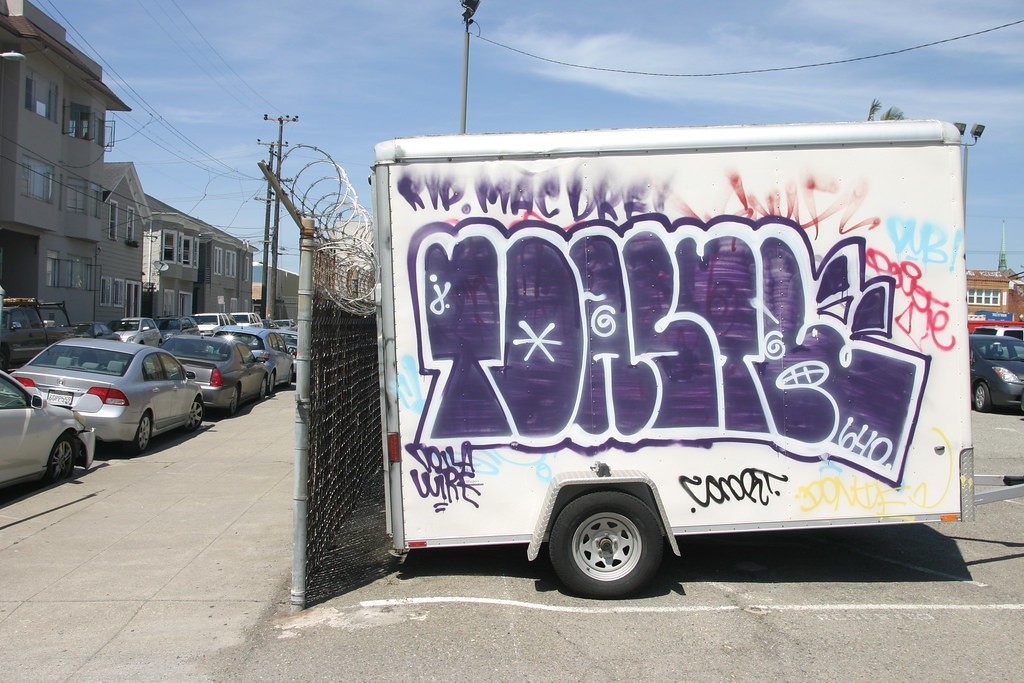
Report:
[10,338,205,452]
[0,369,96,490]
[212,325,294,393]
[0,297,297,375]
[969,334,1024,416]
[158,335,268,416]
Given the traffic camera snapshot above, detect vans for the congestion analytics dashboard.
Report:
[972,326,1024,359]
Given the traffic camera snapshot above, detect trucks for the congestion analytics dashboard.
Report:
[368,121,977,600]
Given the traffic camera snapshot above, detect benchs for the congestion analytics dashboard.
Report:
[56,356,124,373]
